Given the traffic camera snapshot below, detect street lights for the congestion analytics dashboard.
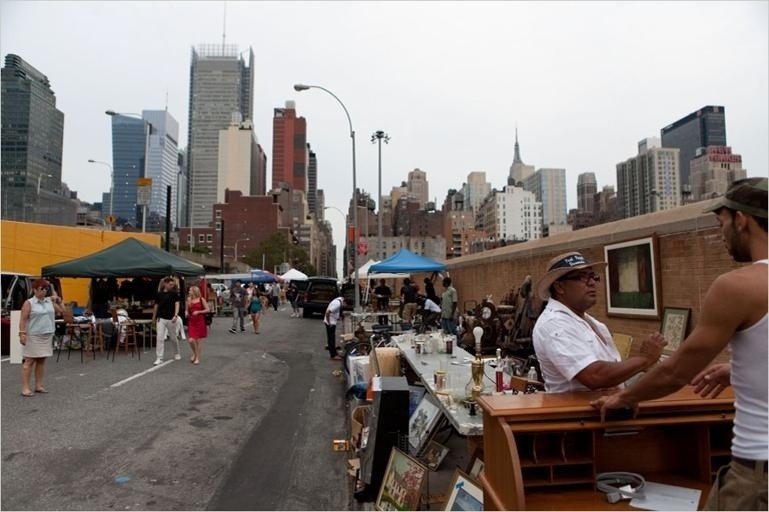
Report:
[104,109,151,233]
[371,129,391,260]
[290,82,364,318]
[322,204,351,282]
[88,158,115,230]
[189,148,196,252]
[360,195,369,265]
[36,172,54,197]
[223,237,251,262]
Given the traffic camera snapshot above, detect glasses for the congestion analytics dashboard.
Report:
[557,270,601,283]
[37,287,48,291]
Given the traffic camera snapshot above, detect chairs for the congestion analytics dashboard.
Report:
[56,307,155,362]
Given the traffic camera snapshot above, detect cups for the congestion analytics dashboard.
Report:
[406,328,457,356]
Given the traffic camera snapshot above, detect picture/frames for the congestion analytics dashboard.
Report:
[659,306,691,356]
[408,391,447,458]
[603,235,662,321]
[374,445,429,511]
[417,440,450,471]
[440,465,484,511]
[465,448,485,485]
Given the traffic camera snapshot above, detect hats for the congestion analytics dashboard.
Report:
[534,249,609,302]
[700,175,769,220]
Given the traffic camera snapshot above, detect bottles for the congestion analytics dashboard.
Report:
[434,370,448,388]
[527,366,539,382]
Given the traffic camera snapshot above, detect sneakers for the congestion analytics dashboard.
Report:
[228,327,238,334]
[253,327,261,334]
[241,328,247,333]
[152,358,165,366]
[193,358,201,365]
[329,353,344,360]
[174,353,182,361]
[296,313,301,318]
[190,352,196,362]
[289,312,296,318]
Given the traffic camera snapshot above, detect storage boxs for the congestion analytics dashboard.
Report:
[345,354,370,373]
[355,359,370,381]
[369,347,401,377]
[408,385,427,418]
[350,404,373,447]
[349,393,372,412]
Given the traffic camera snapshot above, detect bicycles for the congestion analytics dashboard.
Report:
[343,322,429,380]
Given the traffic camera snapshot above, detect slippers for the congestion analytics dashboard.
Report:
[21,389,35,397]
[34,386,49,393]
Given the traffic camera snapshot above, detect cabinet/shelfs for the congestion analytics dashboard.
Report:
[474,382,736,511]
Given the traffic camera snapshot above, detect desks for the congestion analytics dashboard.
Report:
[391,334,528,446]
[351,311,398,337]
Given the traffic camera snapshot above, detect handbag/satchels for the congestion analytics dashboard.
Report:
[203,310,216,326]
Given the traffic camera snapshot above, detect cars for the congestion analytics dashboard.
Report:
[1,271,63,354]
[209,283,231,300]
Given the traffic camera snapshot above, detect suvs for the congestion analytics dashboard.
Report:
[289,277,363,319]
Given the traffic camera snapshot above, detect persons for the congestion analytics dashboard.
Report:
[18,278,66,396]
[205,278,303,335]
[321,276,458,361]
[588,173,768,509]
[152,278,181,365]
[185,285,211,365]
[27,279,58,300]
[531,251,669,392]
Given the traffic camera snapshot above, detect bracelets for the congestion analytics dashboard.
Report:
[57,298,62,304]
[174,315,177,318]
[19,330,26,336]
